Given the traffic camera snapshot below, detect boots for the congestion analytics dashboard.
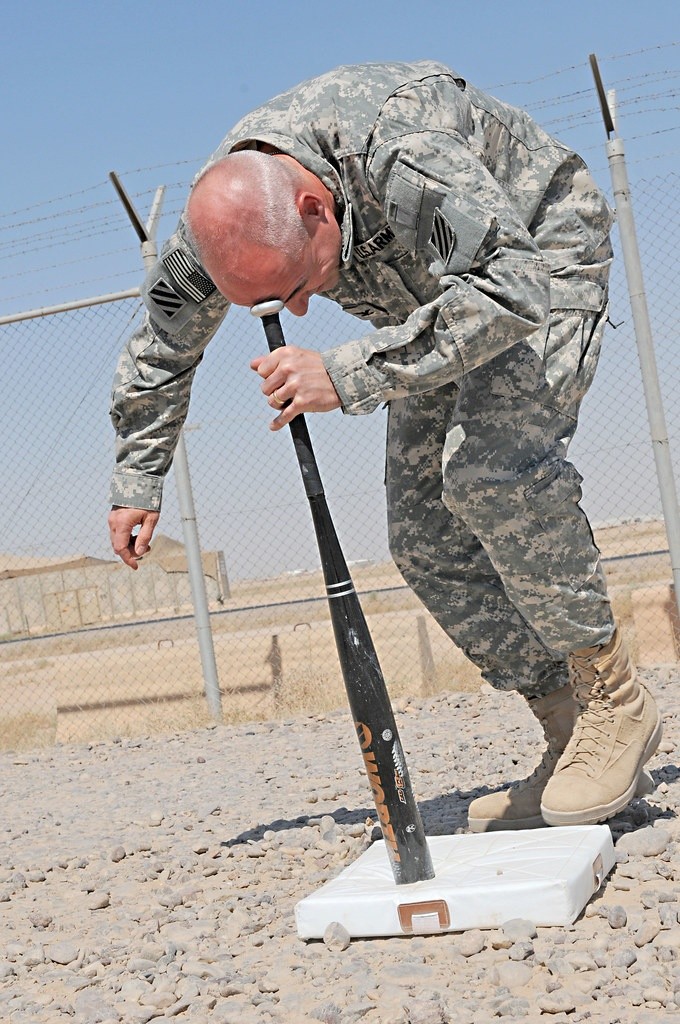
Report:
[541,615,663,826]
[468,681,656,833]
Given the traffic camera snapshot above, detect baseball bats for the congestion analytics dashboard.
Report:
[246,298,442,886]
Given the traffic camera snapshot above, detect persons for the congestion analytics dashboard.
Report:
[106,60,664,832]
